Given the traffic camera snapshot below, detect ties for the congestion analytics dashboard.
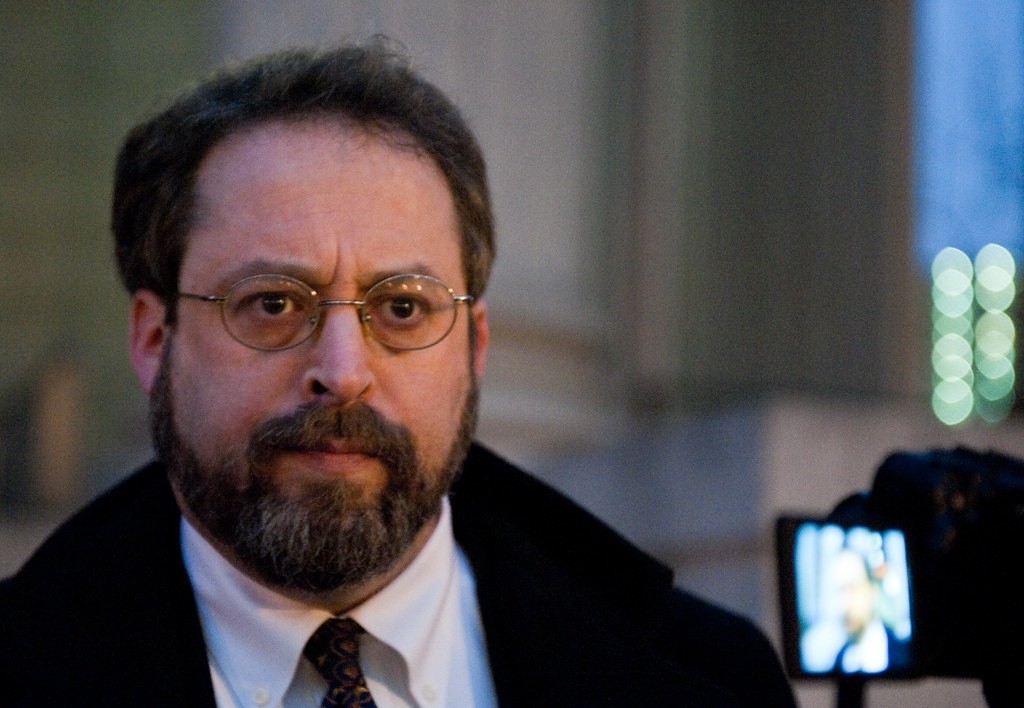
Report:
[301,618,378,708]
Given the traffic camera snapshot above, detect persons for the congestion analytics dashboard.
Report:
[0,34,797,708]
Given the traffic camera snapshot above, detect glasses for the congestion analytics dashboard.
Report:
[161,273,476,354]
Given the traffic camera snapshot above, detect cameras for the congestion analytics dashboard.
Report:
[775,443,1024,708]
